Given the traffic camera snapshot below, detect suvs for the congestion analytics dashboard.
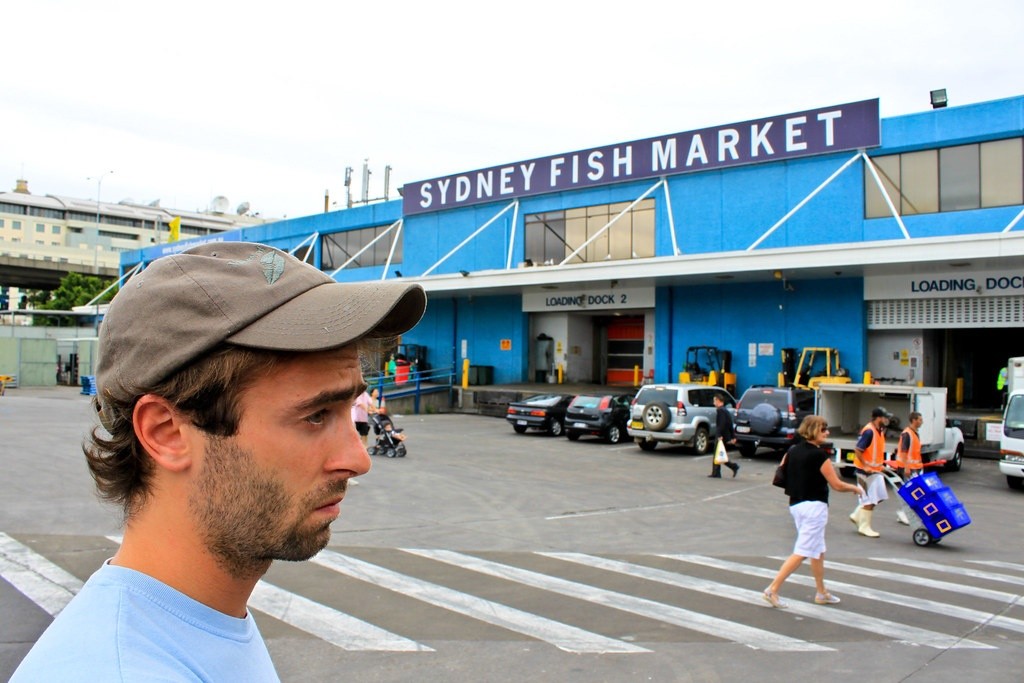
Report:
[626,384,738,456]
[733,384,815,456]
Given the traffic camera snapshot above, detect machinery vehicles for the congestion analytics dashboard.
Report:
[679,346,736,396]
[779,347,852,389]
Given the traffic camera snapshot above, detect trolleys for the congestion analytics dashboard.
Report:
[870,468,942,548]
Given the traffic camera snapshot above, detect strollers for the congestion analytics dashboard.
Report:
[367,412,407,458]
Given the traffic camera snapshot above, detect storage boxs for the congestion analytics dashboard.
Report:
[922,503,971,539]
[911,486,959,521]
[898,472,944,507]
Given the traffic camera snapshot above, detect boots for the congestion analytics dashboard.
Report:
[849,505,879,537]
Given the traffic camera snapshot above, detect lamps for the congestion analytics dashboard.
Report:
[930,88,948,109]
[460,270,469,277]
[523,258,533,266]
[395,270,402,277]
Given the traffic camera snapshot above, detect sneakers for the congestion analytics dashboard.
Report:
[896,510,910,525]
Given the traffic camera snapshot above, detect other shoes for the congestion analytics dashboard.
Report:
[707,473,721,478]
[733,465,739,477]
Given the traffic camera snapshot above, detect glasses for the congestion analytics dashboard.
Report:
[820,428,827,433]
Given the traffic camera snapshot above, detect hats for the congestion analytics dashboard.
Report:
[93,243,426,434]
[872,407,893,418]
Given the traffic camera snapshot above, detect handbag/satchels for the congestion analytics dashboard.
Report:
[713,436,728,466]
[772,465,787,489]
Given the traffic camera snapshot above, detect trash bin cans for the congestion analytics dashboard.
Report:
[478,366,493,385]
[468,365,478,385]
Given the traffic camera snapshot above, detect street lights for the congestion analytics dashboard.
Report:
[87,170,114,335]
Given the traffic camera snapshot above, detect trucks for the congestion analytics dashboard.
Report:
[815,382,964,478]
[1000,357,1023,491]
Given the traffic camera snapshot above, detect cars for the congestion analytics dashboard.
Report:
[564,393,635,445]
[506,394,576,437]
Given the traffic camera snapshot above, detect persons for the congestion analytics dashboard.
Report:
[706,393,741,479]
[367,387,380,443]
[847,406,894,539]
[760,414,864,607]
[996,367,1008,408]
[351,380,381,446]
[7,240,430,683]
[384,421,408,442]
[893,411,925,526]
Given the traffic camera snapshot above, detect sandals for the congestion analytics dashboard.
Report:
[814,592,841,604]
[761,588,788,609]
